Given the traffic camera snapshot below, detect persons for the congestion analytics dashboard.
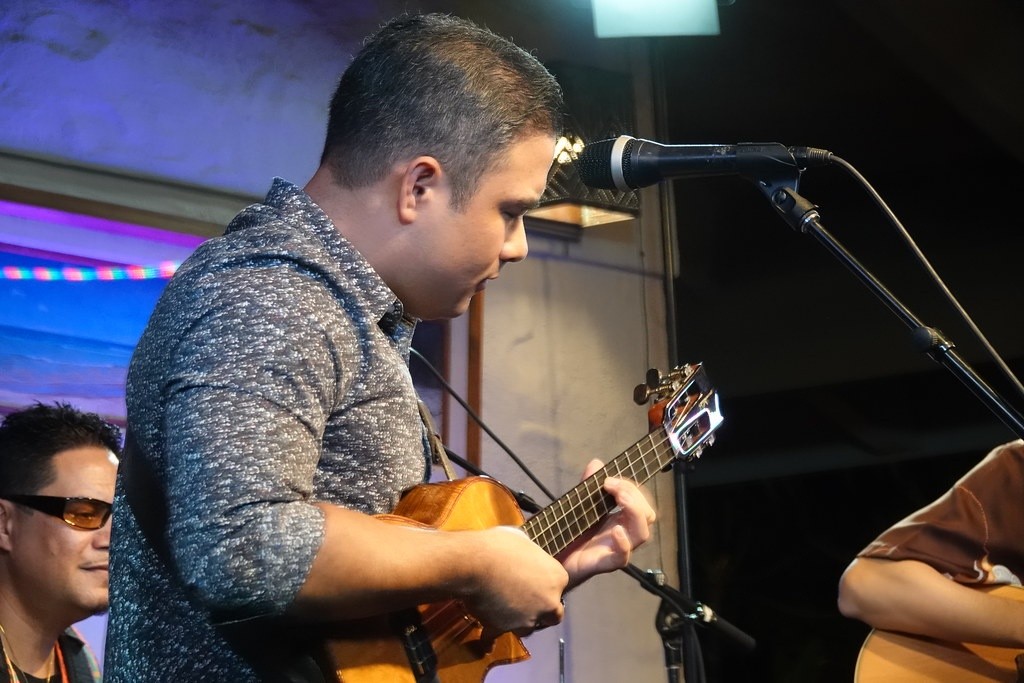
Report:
[0,399,124,682]
[837,436,1024,647]
[103,10,566,683]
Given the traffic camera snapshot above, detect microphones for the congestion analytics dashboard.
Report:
[578,134,809,193]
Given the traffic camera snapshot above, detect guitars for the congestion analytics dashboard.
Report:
[315,359,724,682]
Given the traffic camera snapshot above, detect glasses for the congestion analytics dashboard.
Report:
[0,491,113,530]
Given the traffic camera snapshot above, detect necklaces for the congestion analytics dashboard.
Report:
[0,625,54,683]
